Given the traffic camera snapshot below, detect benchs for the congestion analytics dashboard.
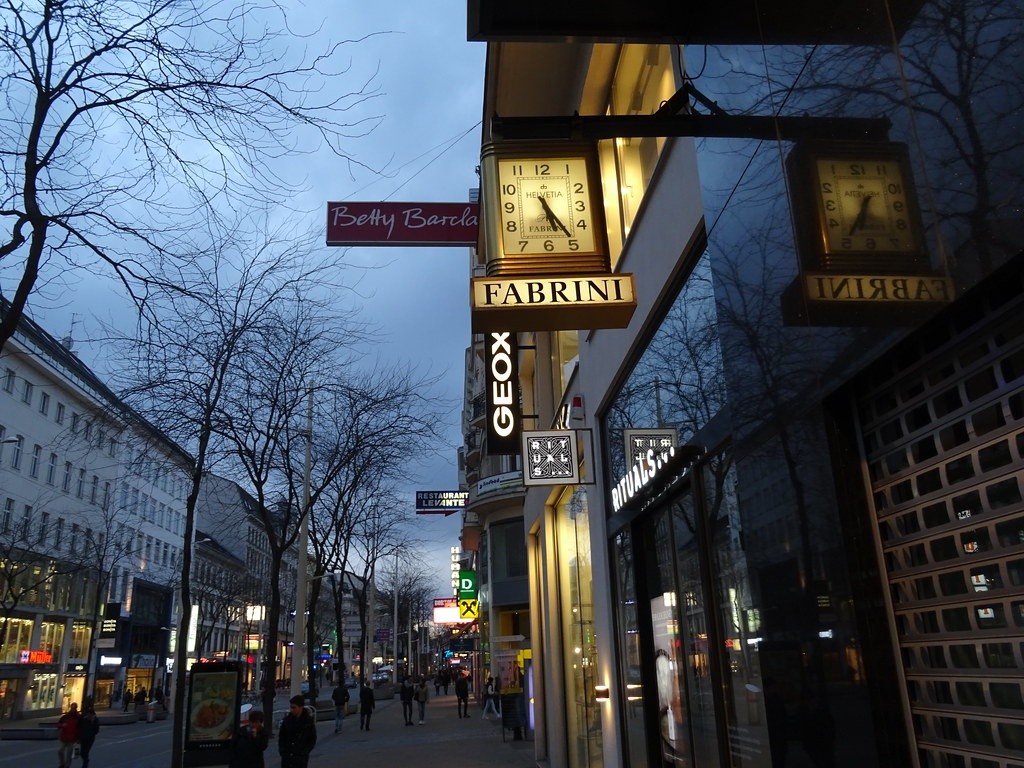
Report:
[297,698,359,722]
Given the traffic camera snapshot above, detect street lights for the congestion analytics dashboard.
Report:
[160,537,211,705]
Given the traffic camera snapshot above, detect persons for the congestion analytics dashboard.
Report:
[123,686,164,712]
[57,699,100,768]
[228,665,502,768]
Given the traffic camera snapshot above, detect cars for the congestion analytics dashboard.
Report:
[345,678,357,689]
[302,681,320,699]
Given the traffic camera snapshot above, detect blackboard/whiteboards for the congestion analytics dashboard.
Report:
[499,693,527,729]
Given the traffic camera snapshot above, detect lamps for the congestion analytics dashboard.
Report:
[595,686,610,703]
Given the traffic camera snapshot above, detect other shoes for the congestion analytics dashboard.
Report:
[463,714,471,718]
[82,759,89,768]
[496,714,502,719]
[405,721,414,727]
[360,726,372,731]
[334,729,343,734]
[481,716,489,720]
[419,720,424,725]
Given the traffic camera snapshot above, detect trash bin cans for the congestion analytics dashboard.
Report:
[146,700,158,722]
[240,704,253,726]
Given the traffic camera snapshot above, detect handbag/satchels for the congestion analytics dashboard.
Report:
[414,687,420,700]
[434,680,436,685]
[72,742,81,759]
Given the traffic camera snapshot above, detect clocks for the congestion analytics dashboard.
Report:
[482,134,613,277]
[783,140,933,276]
